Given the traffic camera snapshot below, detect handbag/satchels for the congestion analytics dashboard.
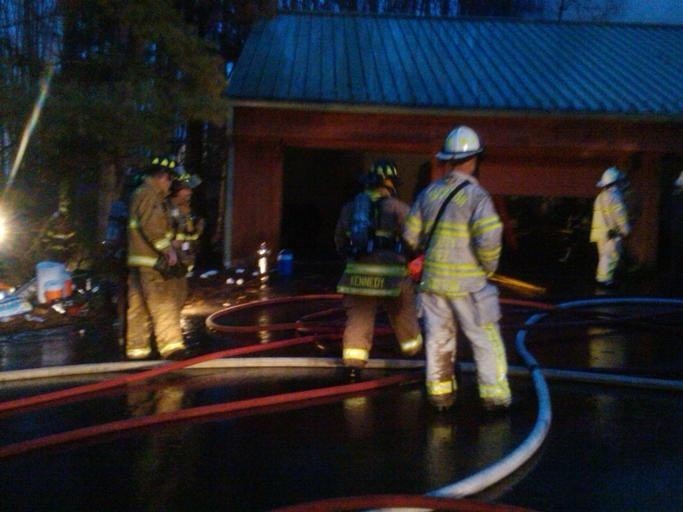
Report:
[409,253,426,283]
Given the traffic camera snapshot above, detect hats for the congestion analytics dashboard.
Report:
[168,165,201,190]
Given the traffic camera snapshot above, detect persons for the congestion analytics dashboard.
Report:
[165,167,205,312]
[402,121,515,412]
[41,208,83,264]
[331,147,426,381]
[587,164,633,297]
[124,152,190,360]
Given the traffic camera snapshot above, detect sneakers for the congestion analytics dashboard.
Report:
[482,398,505,413]
[168,348,193,362]
[342,365,360,382]
[431,397,456,413]
[129,348,161,360]
[600,280,615,287]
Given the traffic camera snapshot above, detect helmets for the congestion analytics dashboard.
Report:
[370,158,398,178]
[596,166,625,187]
[151,152,177,170]
[436,126,484,161]
